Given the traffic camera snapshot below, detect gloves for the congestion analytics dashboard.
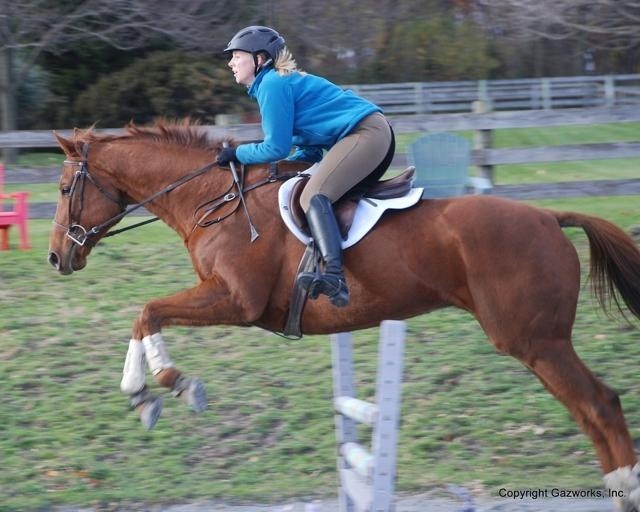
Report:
[217,148,236,167]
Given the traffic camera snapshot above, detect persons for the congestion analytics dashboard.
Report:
[217,26,394,307]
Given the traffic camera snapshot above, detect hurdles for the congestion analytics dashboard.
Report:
[330,320,407,512]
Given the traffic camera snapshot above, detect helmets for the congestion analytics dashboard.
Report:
[223,25,286,61]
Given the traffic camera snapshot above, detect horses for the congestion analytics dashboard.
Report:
[47,115,640,512]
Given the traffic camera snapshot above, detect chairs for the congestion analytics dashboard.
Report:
[407,132,494,199]
[0,162,31,250]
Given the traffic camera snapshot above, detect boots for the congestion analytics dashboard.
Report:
[297,194,350,307]
